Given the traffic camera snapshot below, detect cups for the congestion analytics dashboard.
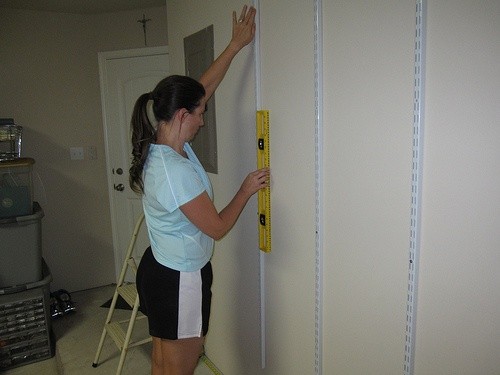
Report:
[10,124,23,160]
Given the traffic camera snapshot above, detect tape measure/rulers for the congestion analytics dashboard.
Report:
[254,110,272,254]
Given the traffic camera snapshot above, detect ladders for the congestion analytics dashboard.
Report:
[92,210,153,375]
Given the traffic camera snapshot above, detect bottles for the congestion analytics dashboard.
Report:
[1,117,16,161]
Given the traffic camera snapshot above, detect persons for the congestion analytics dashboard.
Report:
[130,6,270,374]
